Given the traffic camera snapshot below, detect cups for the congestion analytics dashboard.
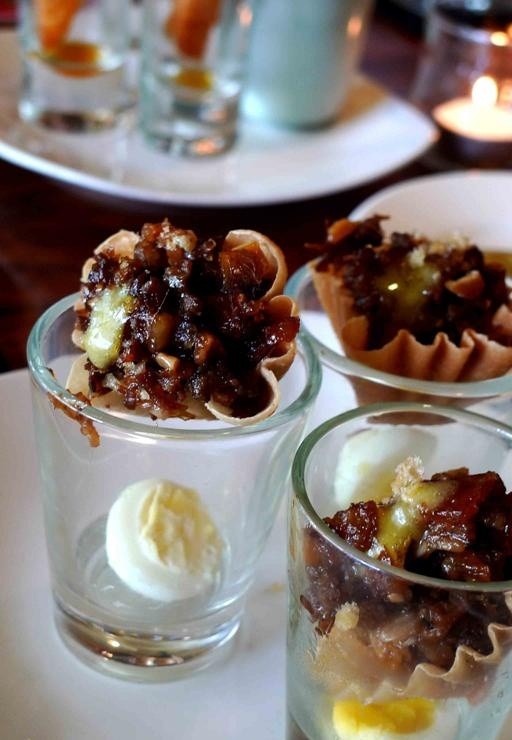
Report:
[281,251,511,487]
[235,1,370,134]
[134,1,256,159]
[27,288,322,685]
[283,400,510,740]
[16,2,136,145]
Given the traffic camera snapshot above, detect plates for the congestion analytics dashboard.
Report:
[1,28,441,209]
[3,306,510,740]
